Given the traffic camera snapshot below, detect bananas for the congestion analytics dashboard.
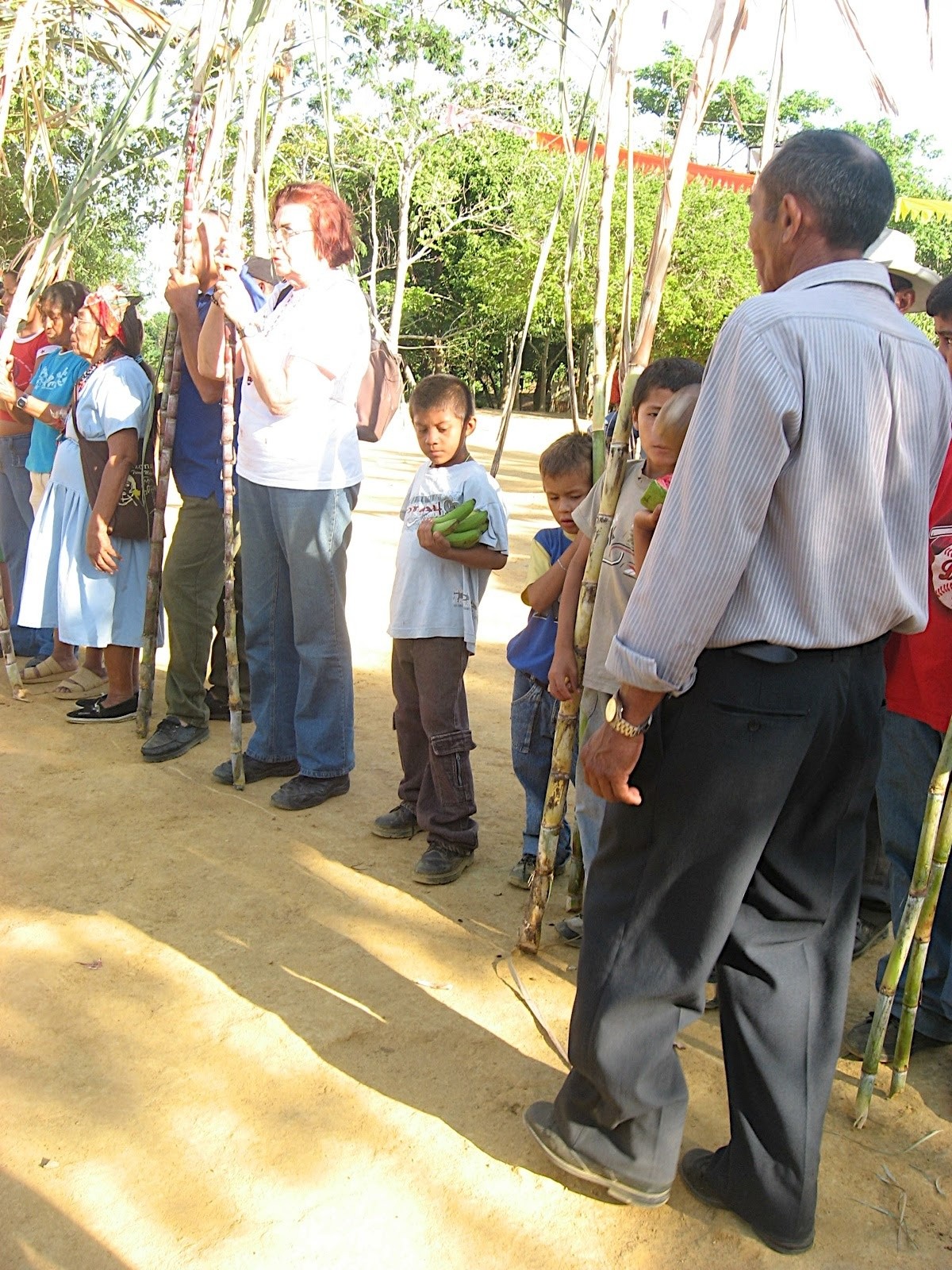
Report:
[430,499,489,549]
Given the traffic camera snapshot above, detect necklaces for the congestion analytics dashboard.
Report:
[76,350,119,393]
[464,453,472,462]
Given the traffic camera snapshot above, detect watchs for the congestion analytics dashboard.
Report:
[16,392,30,410]
[604,687,653,737]
[238,321,263,339]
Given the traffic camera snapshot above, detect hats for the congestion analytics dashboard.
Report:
[861,226,944,312]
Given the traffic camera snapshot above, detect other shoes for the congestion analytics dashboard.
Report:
[75,694,142,711]
[28,653,50,668]
[845,1012,952,1064]
[64,692,140,724]
[372,810,425,841]
[852,921,889,957]
[412,842,476,884]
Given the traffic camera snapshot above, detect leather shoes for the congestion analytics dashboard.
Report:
[680,1147,816,1253]
[522,1101,671,1207]
[201,689,253,722]
[140,716,212,763]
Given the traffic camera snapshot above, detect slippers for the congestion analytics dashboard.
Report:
[52,668,111,700]
[19,657,85,685]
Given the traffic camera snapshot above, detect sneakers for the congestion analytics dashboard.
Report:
[272,770,351,810]
[556,915,584,948]
[210,752,302,784]
[507,853,565,890]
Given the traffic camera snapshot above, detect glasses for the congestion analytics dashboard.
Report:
[73,316,102,330]
[267,226,322,245]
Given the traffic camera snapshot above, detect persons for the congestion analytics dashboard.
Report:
[372,373,511,886]
[196,181,370,811]
[843,224,952,1061]
[507,358,704,949]
[527,129,952,1256]
[0,207,253,763]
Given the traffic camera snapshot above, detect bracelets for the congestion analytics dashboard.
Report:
[211,294,219,307]
[558,559,567,572]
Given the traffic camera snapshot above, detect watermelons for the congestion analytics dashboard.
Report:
[640,474,672,508]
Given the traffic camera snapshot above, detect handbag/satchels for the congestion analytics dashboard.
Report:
[354,338,404,443]
[77,437,166,539]
